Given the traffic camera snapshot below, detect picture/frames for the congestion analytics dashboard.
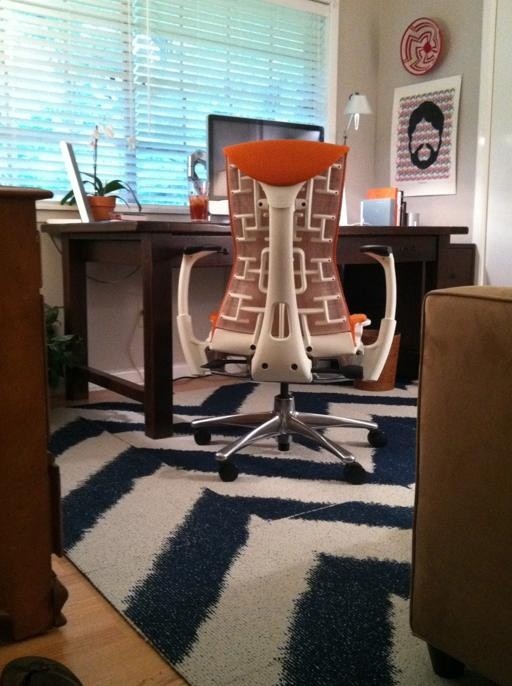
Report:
[390,74,463,196]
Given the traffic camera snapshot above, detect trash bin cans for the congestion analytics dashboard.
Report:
[353,329,401,390]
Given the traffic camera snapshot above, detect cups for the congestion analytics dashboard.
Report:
[406,212,421,228]
[189,178,209,222]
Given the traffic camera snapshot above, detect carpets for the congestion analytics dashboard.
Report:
[45,382,512,686]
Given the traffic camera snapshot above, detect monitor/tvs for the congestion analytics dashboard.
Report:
[207,114,325,202]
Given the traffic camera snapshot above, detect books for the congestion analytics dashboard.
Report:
[360,187,409,226]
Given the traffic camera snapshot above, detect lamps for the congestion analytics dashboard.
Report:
[341,94,373,153]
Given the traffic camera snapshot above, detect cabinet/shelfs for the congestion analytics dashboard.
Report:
[448,244,476,286]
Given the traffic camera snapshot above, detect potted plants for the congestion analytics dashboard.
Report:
[62,173,143,222]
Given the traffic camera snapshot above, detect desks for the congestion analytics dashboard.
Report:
[42,223,469,439]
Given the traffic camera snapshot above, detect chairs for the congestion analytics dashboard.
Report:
[175,142,398,483]
